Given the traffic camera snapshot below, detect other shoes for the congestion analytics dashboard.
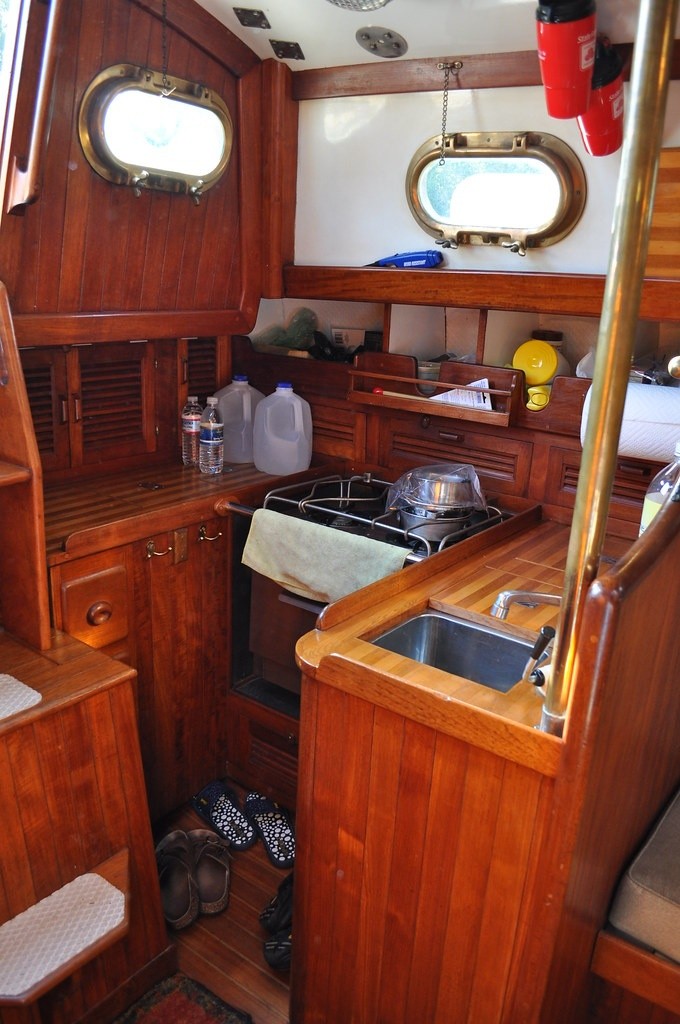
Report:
[264,922,292,968]
[259,872,293,934]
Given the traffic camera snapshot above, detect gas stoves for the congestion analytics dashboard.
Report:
[248,471,517,571]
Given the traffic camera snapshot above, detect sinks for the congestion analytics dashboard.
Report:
[356,597,555,695]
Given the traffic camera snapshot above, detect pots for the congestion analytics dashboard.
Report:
[389,465,479,543]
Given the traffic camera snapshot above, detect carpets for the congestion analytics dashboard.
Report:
[109,972,253,1024]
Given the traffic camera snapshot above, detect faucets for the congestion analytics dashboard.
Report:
[489,589,562,621]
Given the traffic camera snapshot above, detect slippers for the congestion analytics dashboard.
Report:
[191,780,257,852]
[244,790,296,868]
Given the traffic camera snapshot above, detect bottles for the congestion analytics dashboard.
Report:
[199,397,224,473]
[251,383,312,475]
[181,397,203,465]
[213,375,266,463]
[639,442,680,539]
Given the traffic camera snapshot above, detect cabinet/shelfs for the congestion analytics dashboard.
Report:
[50,521,234,828]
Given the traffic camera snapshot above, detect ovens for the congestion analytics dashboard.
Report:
[245,536,413,695]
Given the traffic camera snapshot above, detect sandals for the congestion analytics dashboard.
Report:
[186,829,234,914]
[155,830,200,930]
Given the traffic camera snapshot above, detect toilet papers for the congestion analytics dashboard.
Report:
[580,383,680,463]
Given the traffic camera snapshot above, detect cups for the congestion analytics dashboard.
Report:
[534,0,597,119]
[531,329,564,355]
[512,340,570,383]
[574,42,625,157]
